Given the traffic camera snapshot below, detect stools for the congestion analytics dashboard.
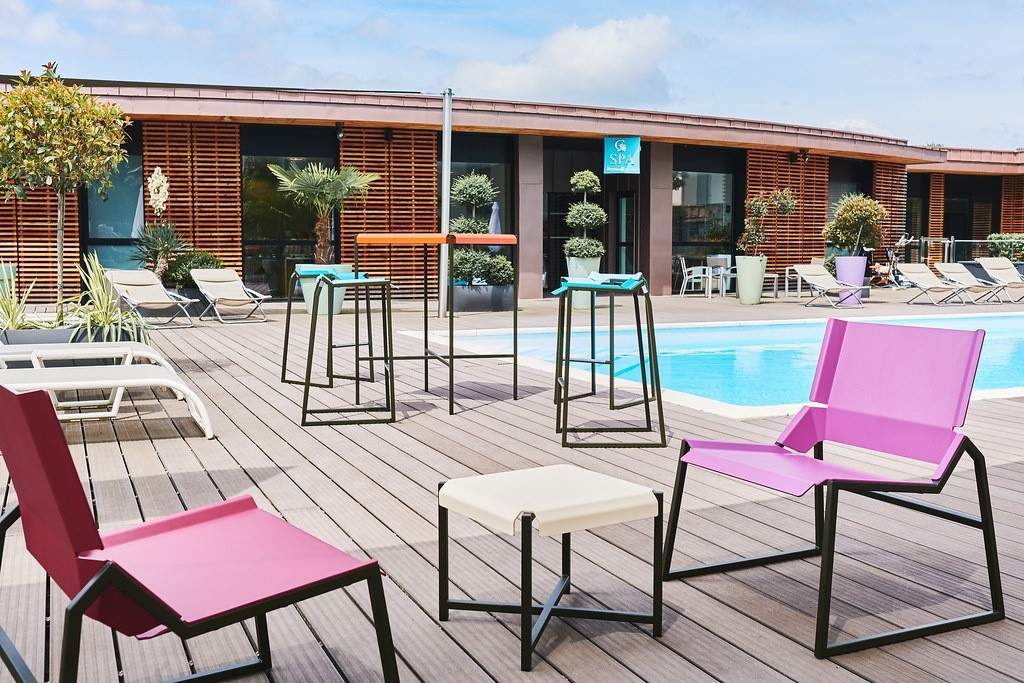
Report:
[280,263,375,388]
[552,279,668,448]
[552,272,655,409]
[301,272,396,426]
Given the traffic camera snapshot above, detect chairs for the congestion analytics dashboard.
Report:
[0,340,186,409]
[792,264,872,308]
[705,256,739,299]
[934,262,1006,304]
[869,264,906,290]
[896,263,971,306]
[662,318,1006,658]
[0,364,213,441]
[973,256,1024,305]
[677,255,713,299]
[785,257,826,298]
[0,384,401,683]
[105,270,200,330]
[189,268,267,325]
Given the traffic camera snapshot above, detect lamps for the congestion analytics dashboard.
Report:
[799,148,809,162]
[336,121,346,139]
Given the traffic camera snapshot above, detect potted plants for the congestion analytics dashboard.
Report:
[735,187,798,304]
[268,160,381,314]
[446,169,515,312]
[563,170,610,310]
[958,232,1024,285]
[129,223,225,317]
[0,62,151,367]
[820,191,890,305]
[822,253,870,297]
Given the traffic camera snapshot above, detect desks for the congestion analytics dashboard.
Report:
[353,232,519,415]
[721,274,779,299]
[437,462,666,672]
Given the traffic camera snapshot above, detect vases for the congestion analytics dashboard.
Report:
[0,265,16,303]
